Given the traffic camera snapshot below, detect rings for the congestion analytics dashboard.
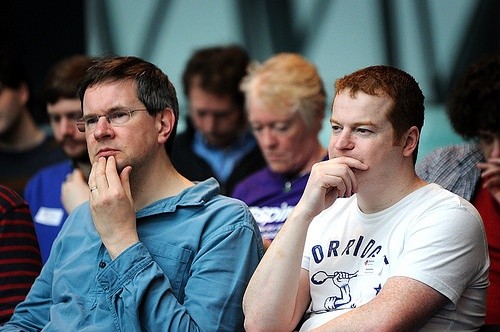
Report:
[90,186,98,192]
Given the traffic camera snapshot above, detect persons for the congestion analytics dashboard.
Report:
[21,57,93,267]
[163,42,268,201]
[230,54,332,256]
[1,184,44,326]
[1,56,264,332]
[414,51,500,332]
[241,73,492,332]
[1,48,69,199]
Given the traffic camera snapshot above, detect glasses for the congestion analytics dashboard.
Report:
[74,106,148,135]
